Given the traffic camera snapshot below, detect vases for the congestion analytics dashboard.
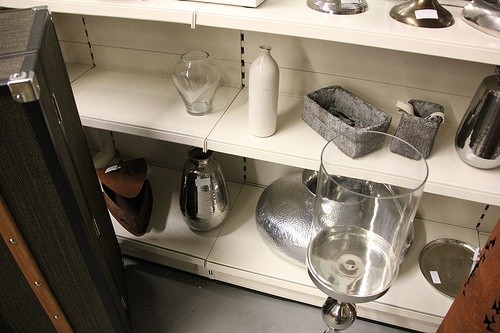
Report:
[249,45,279,137]
[171,49,221,115]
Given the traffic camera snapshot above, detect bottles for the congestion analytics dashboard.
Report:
[171,49,222,116]
[179,148,230,233]
[248,45,280,138]
[454,64,500,169]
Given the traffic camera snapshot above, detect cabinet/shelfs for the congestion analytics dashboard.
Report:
[0,0,500,333]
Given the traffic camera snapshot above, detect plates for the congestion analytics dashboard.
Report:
[417,237,480,301]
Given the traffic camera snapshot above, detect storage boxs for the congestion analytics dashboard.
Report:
[0,6,126,333]
[302,84,392,159]
